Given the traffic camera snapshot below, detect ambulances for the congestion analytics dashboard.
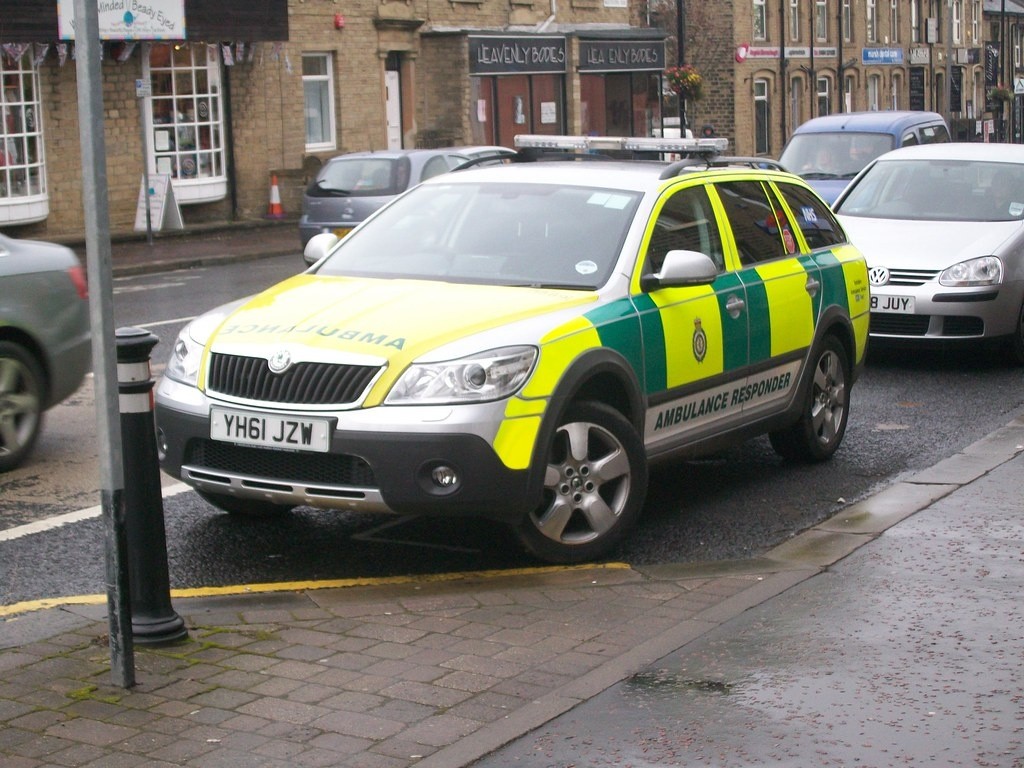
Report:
[152,134,872,568]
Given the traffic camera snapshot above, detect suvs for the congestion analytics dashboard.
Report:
[298,144,518,268]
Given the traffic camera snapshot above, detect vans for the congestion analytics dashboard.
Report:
[770,108,952,210]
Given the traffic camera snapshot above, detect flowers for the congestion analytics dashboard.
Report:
[664,63,703,98]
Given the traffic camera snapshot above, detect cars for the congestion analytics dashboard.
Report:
[0,230,93,476]
[826,142,1024,369]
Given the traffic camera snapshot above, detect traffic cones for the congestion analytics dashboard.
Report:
[260,172,289,220]
